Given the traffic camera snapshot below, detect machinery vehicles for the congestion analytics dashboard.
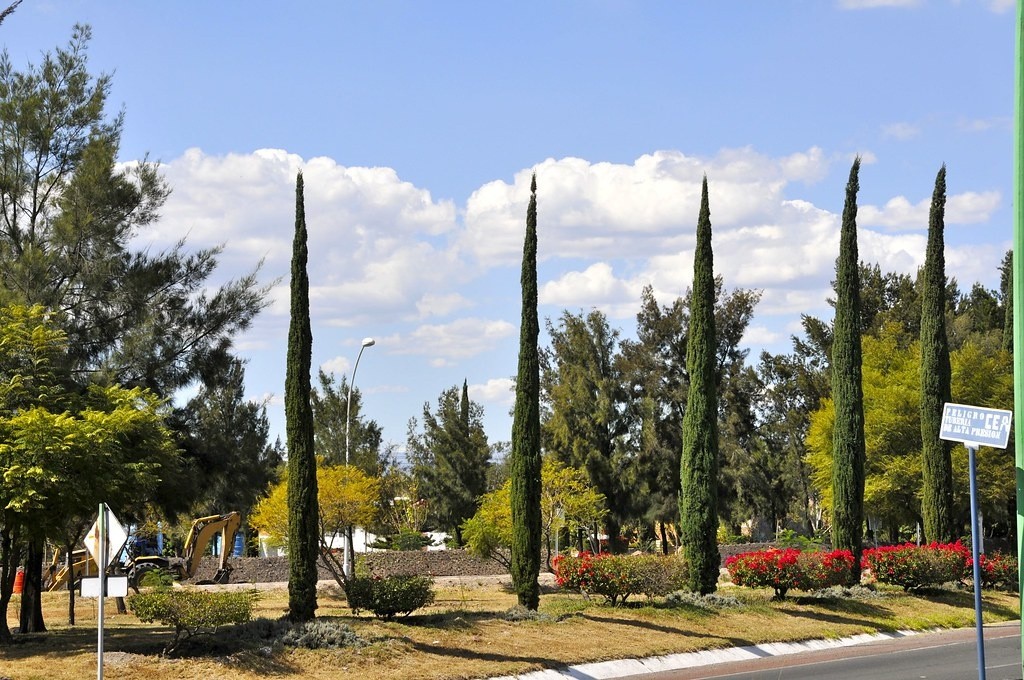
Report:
[43,510,241,590]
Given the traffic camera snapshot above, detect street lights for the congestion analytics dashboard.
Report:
[344,338,377,583]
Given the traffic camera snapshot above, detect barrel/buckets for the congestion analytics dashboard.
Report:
[13,572,23,593]
[233,536,244,557]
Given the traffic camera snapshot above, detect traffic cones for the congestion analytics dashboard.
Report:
[10,571,23,594]
